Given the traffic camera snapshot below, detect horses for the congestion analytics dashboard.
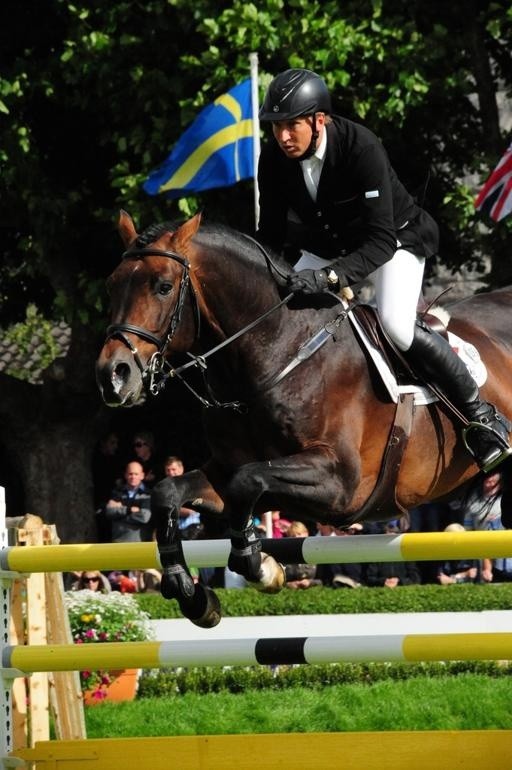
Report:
[94,208,512,631]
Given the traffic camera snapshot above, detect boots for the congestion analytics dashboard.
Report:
[403,319,512,468]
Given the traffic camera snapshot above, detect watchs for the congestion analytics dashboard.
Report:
[322,265,339,292]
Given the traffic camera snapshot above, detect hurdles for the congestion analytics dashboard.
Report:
[1,515,512,770]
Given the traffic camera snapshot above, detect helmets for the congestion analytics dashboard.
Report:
[258,67,330,124]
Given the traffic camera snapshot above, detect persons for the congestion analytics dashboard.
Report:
[64,432,512,590]
[244,68,512,476]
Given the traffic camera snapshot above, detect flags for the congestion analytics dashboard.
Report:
[140,71,256,198]
[468,133,512,233]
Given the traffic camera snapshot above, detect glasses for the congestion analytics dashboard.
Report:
[82,576,98,584]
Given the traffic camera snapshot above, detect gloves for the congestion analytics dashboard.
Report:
[287,267,331,296]
